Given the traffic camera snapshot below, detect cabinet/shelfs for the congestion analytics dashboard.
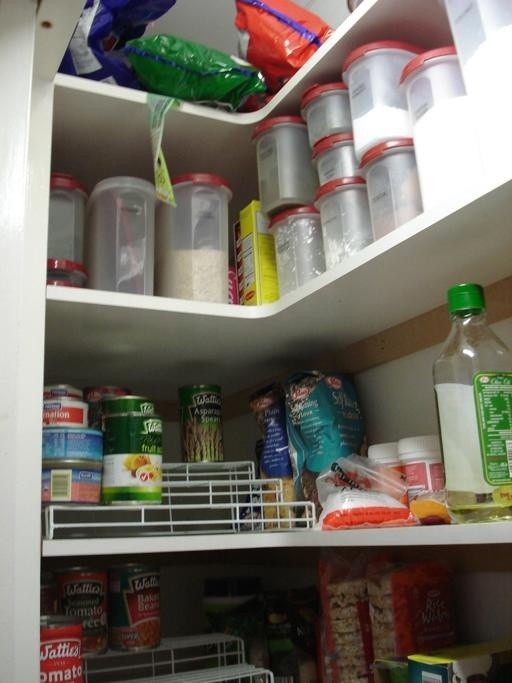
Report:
[42,1,512,556]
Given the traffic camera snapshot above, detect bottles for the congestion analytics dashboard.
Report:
[369,433,445,508]
[432,284,512,523]
[49,173,87,268]
[95,172,234,305]
[254,3,508,294]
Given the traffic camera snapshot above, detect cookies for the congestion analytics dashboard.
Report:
[323,569,412,681]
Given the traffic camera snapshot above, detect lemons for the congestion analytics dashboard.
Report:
[411,497,453,523]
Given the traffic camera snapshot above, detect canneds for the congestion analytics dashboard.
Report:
[41,382,163,507]
[177,383,225,463]
[40,584,65,614]
[54,565,109,656]
[108,561,162,652]
[40,616,86,683]
